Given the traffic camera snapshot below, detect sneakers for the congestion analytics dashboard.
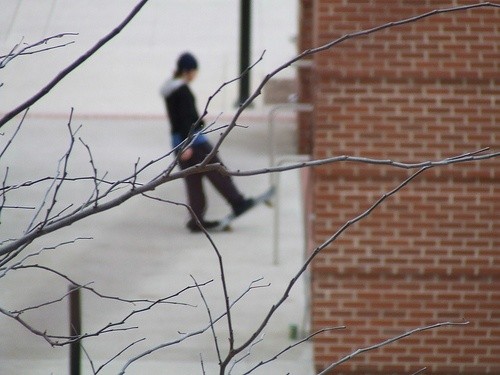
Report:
[193,222,218,230]
[233,199,253,215]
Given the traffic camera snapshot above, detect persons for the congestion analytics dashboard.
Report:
[160,52,255,234]
